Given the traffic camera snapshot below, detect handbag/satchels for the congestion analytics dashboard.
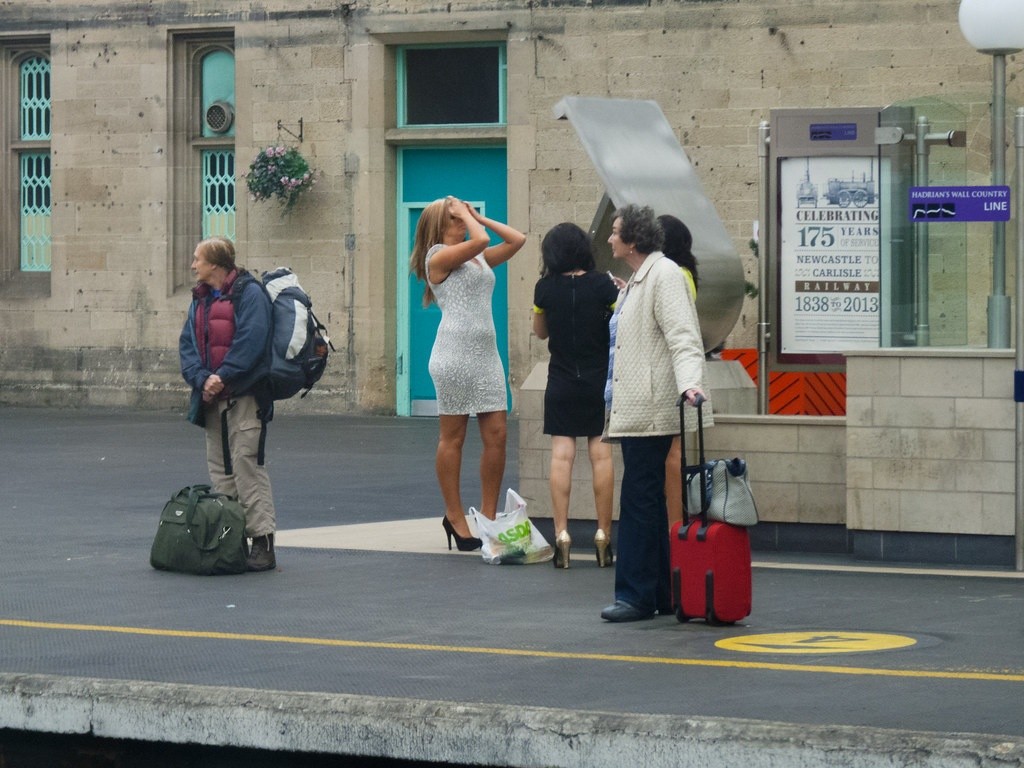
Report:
[148,483,245,575]
[683,458,760,527]
[468,487,554,565]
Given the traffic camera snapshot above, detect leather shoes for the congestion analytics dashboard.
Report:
[599,598,655,621]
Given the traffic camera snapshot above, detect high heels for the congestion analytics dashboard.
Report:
[593,528,613,568]
[551,530,571,568]
[441,515,482,551]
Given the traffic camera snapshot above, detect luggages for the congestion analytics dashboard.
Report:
[669,391,752,624]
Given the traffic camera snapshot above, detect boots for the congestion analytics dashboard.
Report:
[246,532,275,571]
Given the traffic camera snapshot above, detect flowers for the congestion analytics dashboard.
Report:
[240,145,277,203]
[274,144,320,220]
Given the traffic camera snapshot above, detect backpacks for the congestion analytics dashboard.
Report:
[252,265,335,401]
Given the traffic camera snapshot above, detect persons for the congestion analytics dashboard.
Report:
[180,235,282,573]
[533,221,626,573]
[599,204,708,627]
[607,215,702,535]
[409,195,527,555]
